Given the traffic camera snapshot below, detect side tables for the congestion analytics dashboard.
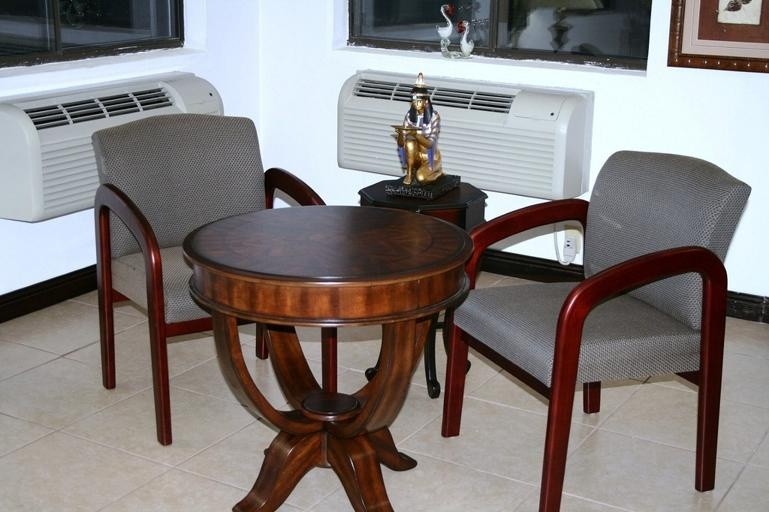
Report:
[357,180,487,398]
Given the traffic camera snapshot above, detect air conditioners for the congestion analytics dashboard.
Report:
[0,71,219,223]
[336,71,594,200]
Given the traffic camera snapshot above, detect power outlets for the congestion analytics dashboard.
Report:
[562,224,581,260]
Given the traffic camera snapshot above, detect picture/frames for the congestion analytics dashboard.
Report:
[666,0,769,73]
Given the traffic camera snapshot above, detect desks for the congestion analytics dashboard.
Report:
[180,205,474,512]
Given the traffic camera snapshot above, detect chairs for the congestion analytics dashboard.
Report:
[440,150,751,512]
[91,112,328,448]
